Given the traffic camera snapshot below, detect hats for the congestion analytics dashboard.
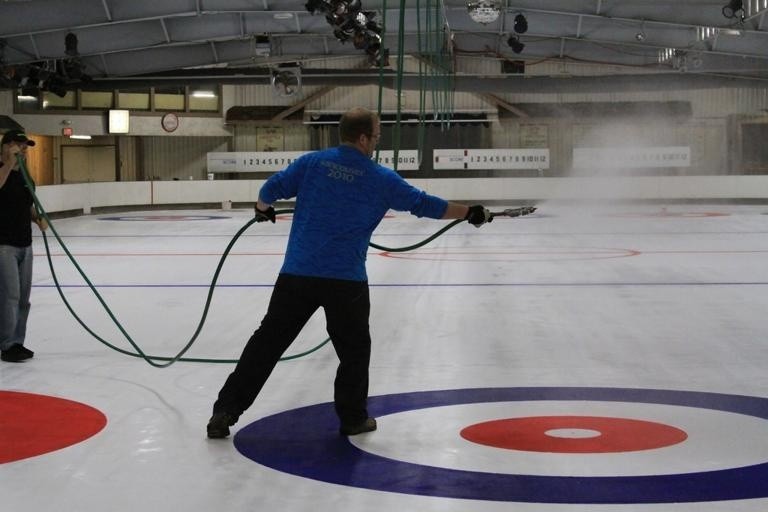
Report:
[1,129,36,147]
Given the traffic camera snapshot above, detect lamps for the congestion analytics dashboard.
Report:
[635,18,649,43]
[507,10,529,53]
[466,1,501,28]
[721,1,747,23]
[305,0,389,68]
[61,29,80,58]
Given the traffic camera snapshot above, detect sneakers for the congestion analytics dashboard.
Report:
[340,416,378,438]
[0,342,35,363]
[206,411,234,438]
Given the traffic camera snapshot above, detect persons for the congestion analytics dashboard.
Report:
[0,129,49,362]
[206,106,493,439]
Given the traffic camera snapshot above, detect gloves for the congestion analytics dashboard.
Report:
[253,202,276,224]
[464,204,492,230]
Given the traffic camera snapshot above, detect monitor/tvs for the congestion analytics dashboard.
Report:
[108,108,130,134]
